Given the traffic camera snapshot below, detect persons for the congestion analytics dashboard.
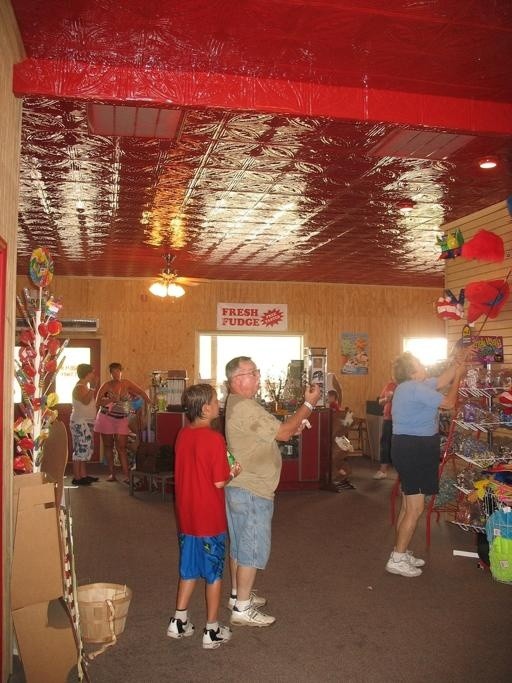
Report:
[224,354,323,629]
[381,351,467,579]
[95,362,153,483]
[371,367,396,480]
[167,383,243,650]
[68,363,102,487]
[328,389,341,412]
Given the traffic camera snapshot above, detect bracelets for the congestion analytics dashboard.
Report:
[303,400,315,411]
[230,473,235,478]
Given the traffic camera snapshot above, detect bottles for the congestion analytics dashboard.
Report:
[458,359,497,422]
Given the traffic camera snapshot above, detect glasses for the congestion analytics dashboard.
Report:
[234,369,260,377]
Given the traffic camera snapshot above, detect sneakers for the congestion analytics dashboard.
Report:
[227,588,267,611]
[105,474,116,481]
[202,624,232,650]
[230,603,276,627]
[72,479,91,485]
[166,617,194,639]
[390,551,426,567]
[83,476,100,482]
[373,471,386,479]
[385,559,423,577]
[123,478,129,483]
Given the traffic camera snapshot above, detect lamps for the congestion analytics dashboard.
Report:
[149,253,186,299]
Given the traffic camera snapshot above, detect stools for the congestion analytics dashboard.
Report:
[129,470,173,505]
